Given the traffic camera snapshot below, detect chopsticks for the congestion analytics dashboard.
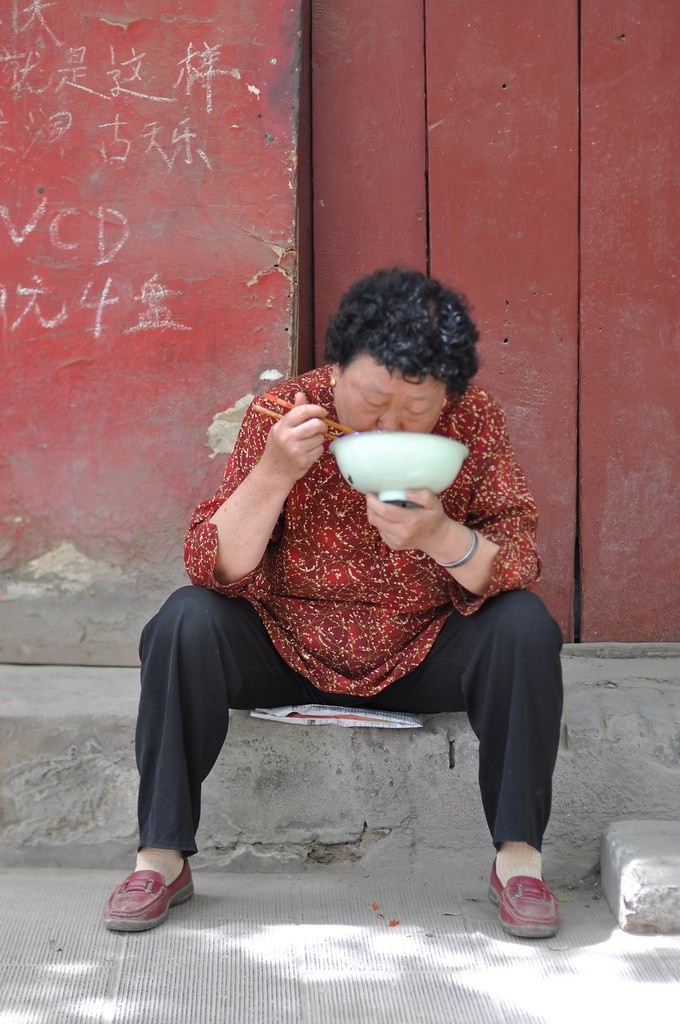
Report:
[254,394,354,441]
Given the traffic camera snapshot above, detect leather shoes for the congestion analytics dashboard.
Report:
[102,856,194,931]
[487,857,561,938]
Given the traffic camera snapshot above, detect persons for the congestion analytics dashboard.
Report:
[102,262,564,938]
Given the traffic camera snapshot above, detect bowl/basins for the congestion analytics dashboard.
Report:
[329,431,470,507]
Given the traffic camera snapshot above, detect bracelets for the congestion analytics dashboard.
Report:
[436,528,478,569]
